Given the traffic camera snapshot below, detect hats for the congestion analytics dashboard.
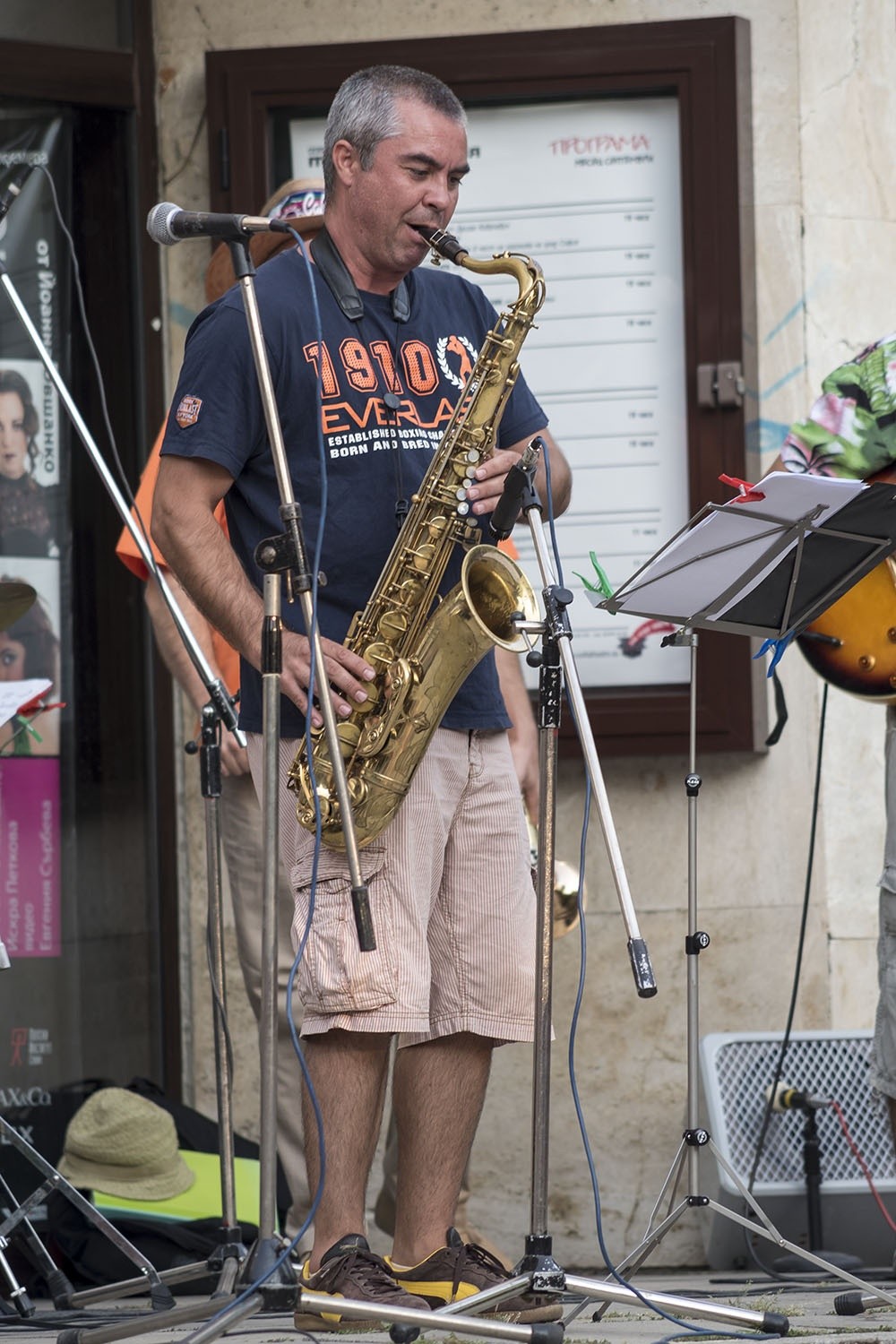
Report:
[204,177,332,305]
[58,1086,197,1200]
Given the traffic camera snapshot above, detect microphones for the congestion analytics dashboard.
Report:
[764,1084,835,1112]
[488,436,542,542]
[146,202,292,247]
[0,165,34,224]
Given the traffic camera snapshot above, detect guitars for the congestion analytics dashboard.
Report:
[790,458,896,705]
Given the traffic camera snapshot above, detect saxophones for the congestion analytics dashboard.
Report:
[282,225,552,854]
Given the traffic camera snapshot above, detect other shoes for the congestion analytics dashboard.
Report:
[298,1252,310,1263]
[452,1227,516,1272]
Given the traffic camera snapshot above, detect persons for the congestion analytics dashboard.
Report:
[115,65,572,1331]
[0,369,59,558]
[0,575,61,757]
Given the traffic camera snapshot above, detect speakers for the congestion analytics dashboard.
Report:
[688,1029,896,1282]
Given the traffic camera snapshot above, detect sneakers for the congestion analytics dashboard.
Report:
[293,1233,431,1330]
[383,1228,565,1324]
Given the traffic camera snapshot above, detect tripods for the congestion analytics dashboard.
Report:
[0,236,895,1344]
